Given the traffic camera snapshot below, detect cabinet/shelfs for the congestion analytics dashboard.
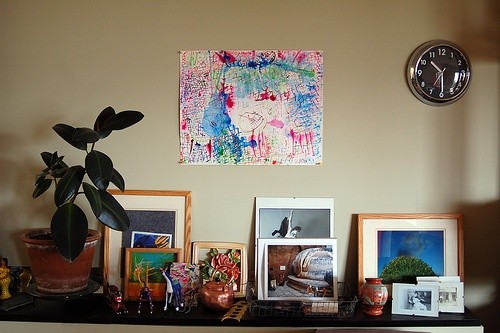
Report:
[0,264,485,333]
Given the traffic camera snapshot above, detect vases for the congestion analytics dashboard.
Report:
[359,277,388,315]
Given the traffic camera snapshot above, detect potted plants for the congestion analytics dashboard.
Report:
[22,106,145,298]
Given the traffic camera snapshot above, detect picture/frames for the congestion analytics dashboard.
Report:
[256,237,337,300]
[99,188,193,302]
[255,196,334,287]
[419,280,465,312]
[357,213,466,300]
[190,240,248,298]
[415,275,461,281]
[122,246,182,300]
[392,282,440,316]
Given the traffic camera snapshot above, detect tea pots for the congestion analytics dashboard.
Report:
[200,265,234,311]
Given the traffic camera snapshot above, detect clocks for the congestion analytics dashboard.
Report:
[406,40,472,107]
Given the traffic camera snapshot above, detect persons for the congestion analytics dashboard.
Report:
[285,210,301,238]
[0,256,12,300]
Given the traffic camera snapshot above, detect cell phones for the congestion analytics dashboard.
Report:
[0,293,34,313]
